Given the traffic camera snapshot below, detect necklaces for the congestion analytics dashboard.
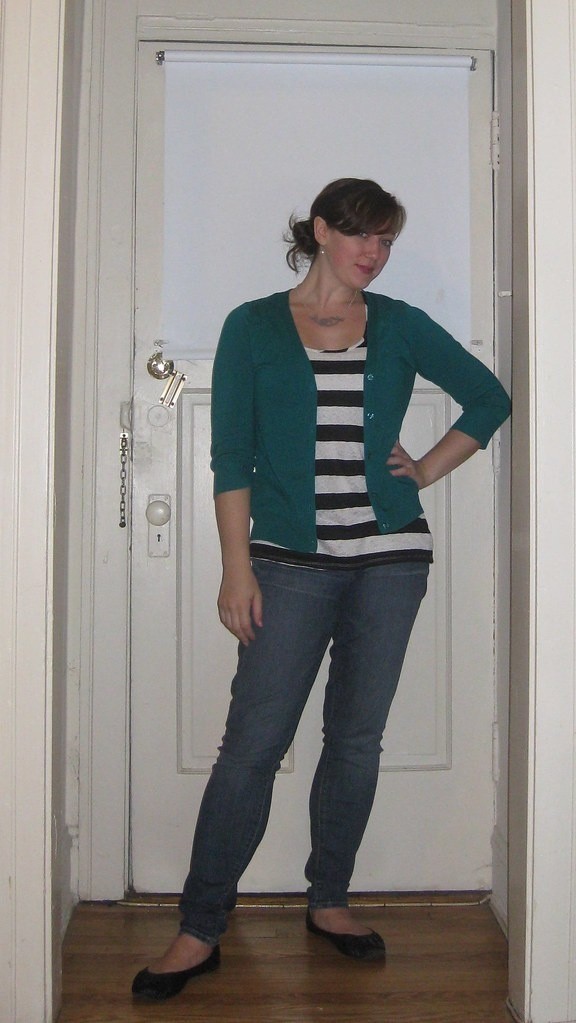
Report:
[297,283,358,325]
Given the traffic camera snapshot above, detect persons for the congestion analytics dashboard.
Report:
[132,178,512,1000]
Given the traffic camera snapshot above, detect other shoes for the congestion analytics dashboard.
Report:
[306,906,386,961]
[132,944,221,1000]
